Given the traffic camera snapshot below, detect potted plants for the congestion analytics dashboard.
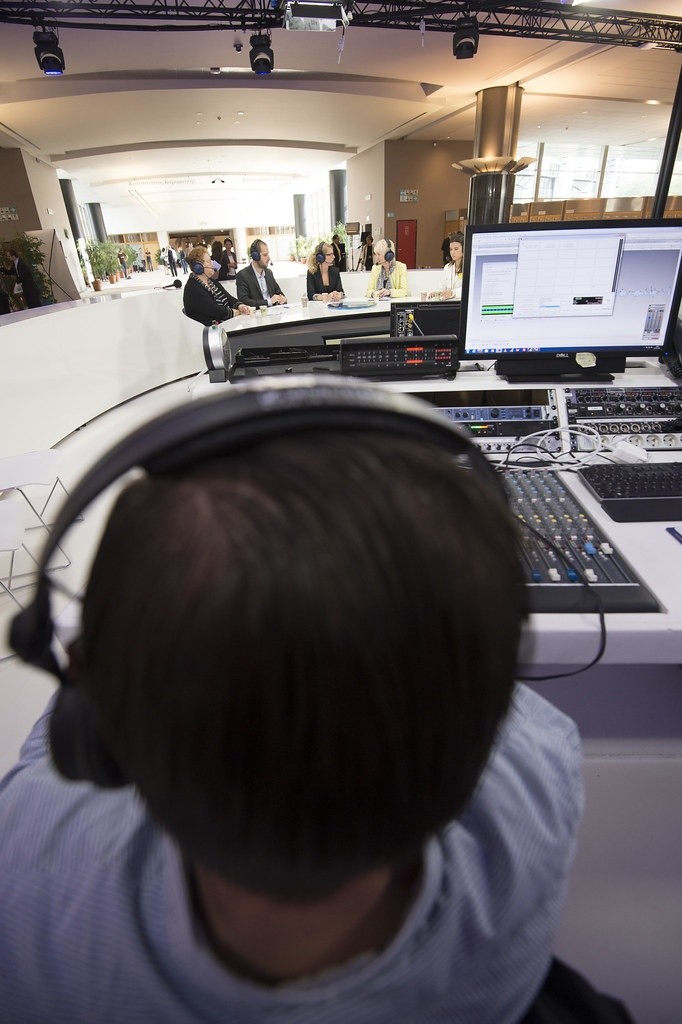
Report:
[288,236,314,264]
[77,241,138,291]
[154,249,165,269]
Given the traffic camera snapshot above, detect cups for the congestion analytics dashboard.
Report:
[249,306,257,316]
[373,292,380,305]
[258,305,268,317]
[301,297,307,308]
[420,292,428,302]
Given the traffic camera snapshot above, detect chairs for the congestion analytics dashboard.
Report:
[180,307,209,392]
[0,447,83,659]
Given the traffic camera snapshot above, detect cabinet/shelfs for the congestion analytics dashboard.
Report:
[460,196,682,232]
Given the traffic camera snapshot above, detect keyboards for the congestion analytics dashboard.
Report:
[577,461,682,504]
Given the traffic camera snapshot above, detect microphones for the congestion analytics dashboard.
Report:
[376,260,390,266]
[324,260,335,264]
[270,260,273,266]
[204,266,217,272]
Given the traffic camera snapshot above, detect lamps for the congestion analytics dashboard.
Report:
[33,26,66,76]
[212,178,225,183]
[250,30,274,75]
[452,15,480,59]
[450,155,536,176]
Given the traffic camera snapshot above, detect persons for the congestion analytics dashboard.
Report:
[0,250,42,309]
[330,235,344,272]
[427,231,464,301]
[355,235,375,271]
[117,248,129,278]
[209,238,238,280]
[365,239,410,298]
[161,246,188,277]
[137,247,146,272]
[236,238,287,310]
[183,247,250,326]
[307,242,345,302]
[144,248,153,272]
[0,426,583,1024]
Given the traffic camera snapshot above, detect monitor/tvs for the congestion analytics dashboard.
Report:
[457,217,682,382]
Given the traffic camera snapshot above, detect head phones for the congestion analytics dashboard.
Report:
[252,239,262,260]
[316,242,326,263]
[385,238,394,261]
[8,374,513,790]
[185,257,204,275]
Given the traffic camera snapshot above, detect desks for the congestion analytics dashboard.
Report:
[218,294,433,331]
